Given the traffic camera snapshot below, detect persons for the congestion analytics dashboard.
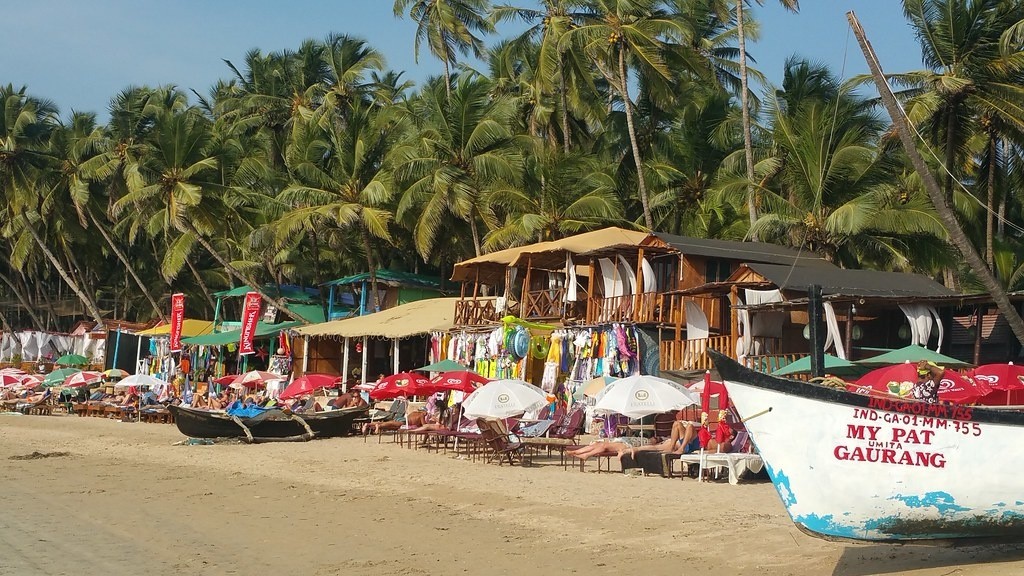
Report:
[567,436,659,462]
[666,422,736,455]
[2,387,366,423]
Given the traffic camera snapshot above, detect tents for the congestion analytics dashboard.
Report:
[135,319,221,373]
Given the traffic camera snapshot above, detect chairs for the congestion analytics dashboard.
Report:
[360,395,764,485]
[1,378,359,428]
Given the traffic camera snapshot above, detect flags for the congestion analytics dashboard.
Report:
[170,292,184,352]
[239,292,260,355]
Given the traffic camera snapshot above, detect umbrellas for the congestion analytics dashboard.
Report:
[771,337,1023,404]
[352,357,557,451]
[597,372,701,447]
[1,356,339,397]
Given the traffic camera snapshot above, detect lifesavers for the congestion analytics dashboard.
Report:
[502,327,530,360]
[531,336,550,360]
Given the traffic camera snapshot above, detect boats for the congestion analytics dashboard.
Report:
[162,399,368,442]
[702,346,1024,543]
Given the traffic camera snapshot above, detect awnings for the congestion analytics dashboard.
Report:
[660,280,772,296]
[182,320,303,345]
[293,295,515,342]
[577,244,670,257]
